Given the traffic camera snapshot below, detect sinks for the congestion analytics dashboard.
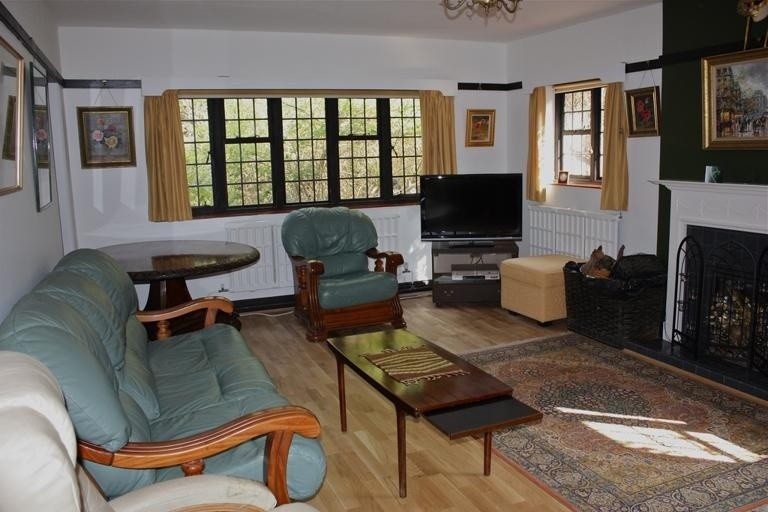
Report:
[1,249,328,502]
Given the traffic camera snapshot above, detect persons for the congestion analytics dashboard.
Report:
[716,113,766,137]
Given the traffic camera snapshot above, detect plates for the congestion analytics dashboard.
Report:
[433,241,519,307]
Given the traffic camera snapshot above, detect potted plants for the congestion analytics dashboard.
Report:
[701,48,768,150]
[2,95,15,161]
[34,105,50,169]
[624,86,661,137]
[76,107,136,169]
[465,108,496,147]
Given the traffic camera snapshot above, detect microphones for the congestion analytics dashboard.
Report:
[500,254,589,328]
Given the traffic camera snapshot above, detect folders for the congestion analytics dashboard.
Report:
[218,213,412,293]
[528,204,624,261]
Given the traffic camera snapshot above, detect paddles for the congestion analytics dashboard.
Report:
[457,332,768,512]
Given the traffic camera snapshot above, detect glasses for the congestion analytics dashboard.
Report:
[443,0,523,17]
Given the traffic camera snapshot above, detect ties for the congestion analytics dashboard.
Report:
[420,173,523,248]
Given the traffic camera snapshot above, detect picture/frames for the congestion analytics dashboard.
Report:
[563,252,667,352]
[97,239,260,341]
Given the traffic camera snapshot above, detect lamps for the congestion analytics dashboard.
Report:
[1,350,319,512]
[281,206,408,343]
[0,350,321,512]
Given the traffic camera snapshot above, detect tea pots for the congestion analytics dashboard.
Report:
[30,60,53,213]
[0,36,25,199]
